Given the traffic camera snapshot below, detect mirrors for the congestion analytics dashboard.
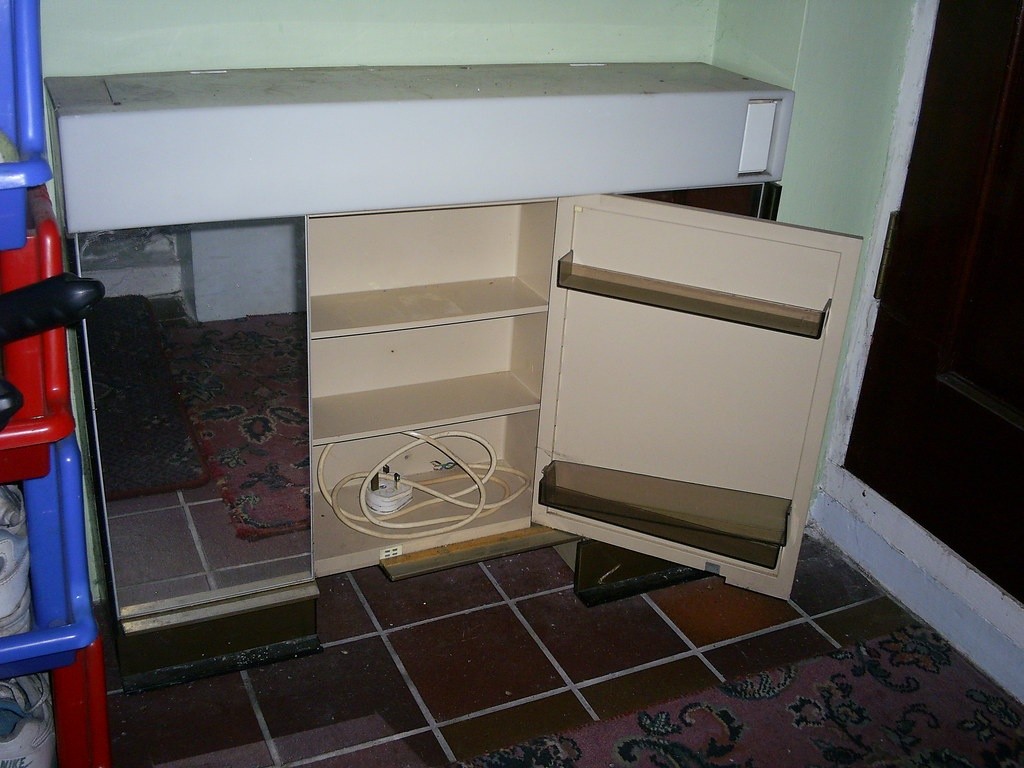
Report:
[76,213,314,621]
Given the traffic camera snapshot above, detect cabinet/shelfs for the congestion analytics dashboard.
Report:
[306,192,863,605]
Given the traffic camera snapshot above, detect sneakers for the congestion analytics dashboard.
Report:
[0,484,34,636]
[0,678,54,767]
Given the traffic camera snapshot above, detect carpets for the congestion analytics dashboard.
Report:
[447,624,1024,768]
[161,310,311,545]
[86,293,210,503]
